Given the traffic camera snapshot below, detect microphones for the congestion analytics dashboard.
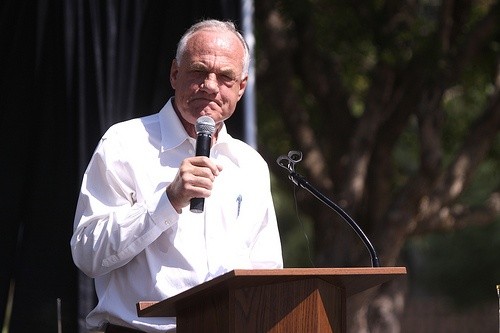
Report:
[190,116,215,213]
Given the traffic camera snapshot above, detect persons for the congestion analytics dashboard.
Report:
[71,20,283,333]
